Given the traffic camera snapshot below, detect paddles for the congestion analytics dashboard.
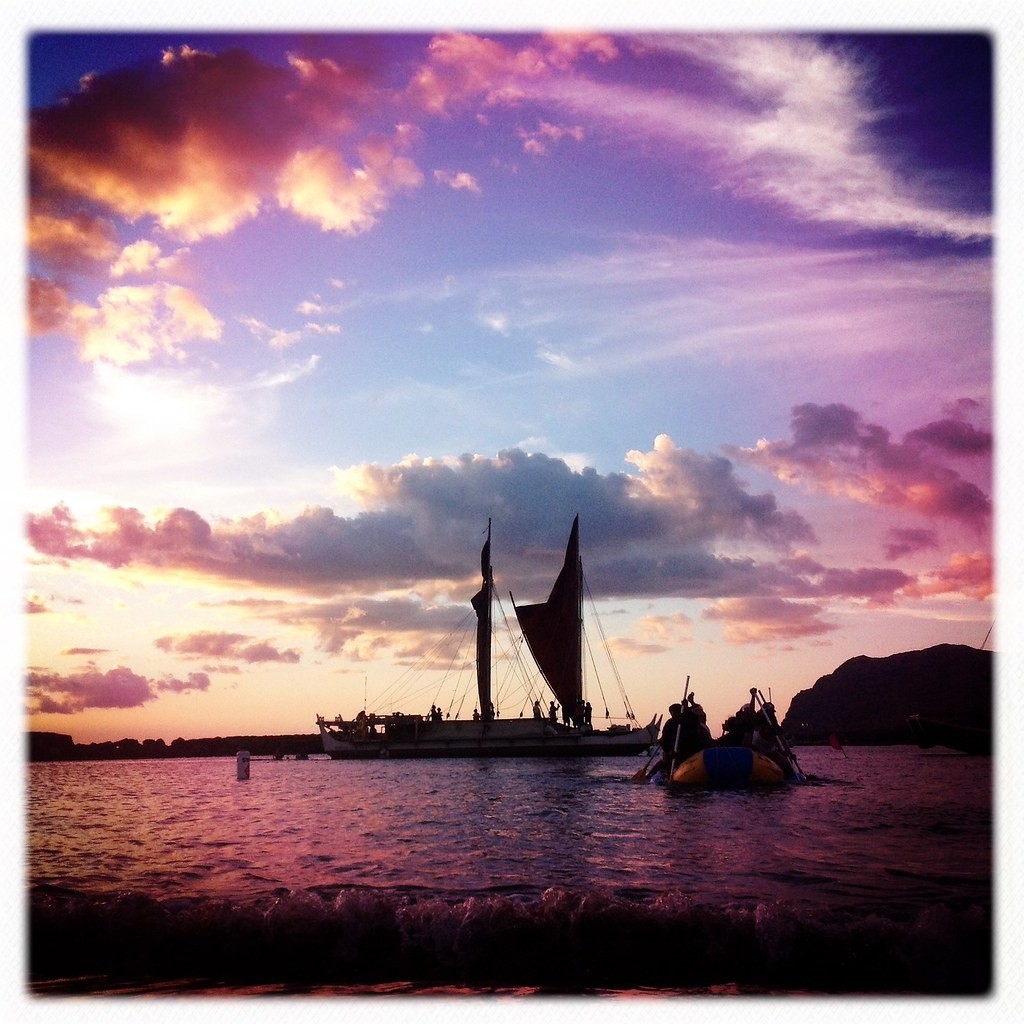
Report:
[758,689,803,773]
[632,691,695,782]
[755,693,807,782]
[670,674,690,779]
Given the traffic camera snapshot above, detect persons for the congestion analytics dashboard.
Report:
[431,700,592,729]
[658,688,797,768]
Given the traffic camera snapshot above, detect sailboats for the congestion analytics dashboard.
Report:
[316,513,665,757]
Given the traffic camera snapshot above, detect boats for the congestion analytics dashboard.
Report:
[904,712,991,757]
[668,745,786,794]
